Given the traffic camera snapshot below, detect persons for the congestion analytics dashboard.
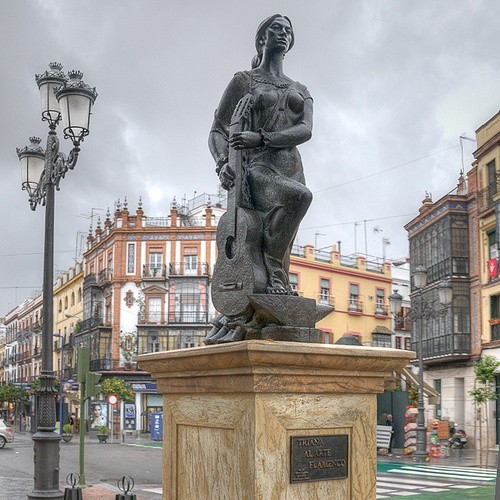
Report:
[90,404,107,428]
[385,414,396,455]
[208,14,314,294]
[69,417,73,431]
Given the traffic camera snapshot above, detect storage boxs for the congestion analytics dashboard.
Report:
[405,408,418,453]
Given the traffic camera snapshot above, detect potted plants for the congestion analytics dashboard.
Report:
[62,424,73,444]
[98,427,110,443]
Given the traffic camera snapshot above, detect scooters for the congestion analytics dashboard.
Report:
[449,423,467,461]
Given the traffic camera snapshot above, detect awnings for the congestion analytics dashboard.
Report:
[395,368,441,404]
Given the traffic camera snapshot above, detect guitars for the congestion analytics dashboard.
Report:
[211,93,269,316]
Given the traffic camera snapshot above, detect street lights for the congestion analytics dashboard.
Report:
[14,61,99,500]
[53,333,63,435]
[15,353,24,431]
[387,262,453,463]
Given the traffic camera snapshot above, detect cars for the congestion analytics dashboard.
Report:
[0,419,14,449]
